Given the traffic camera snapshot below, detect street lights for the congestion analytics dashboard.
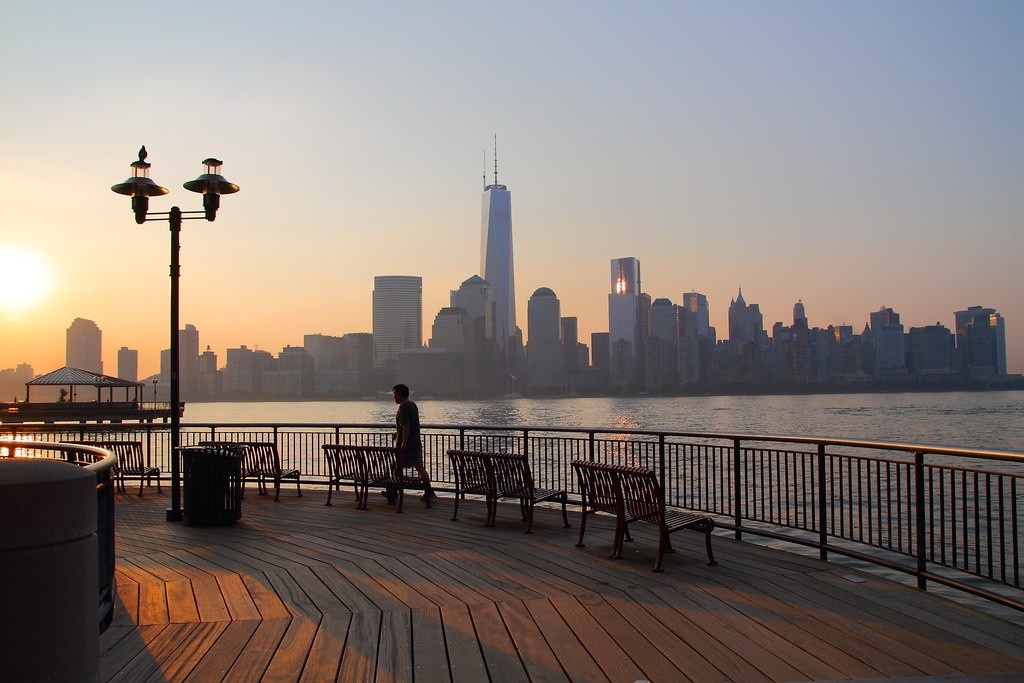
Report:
[152,379,158,409]
[111,144,240,525]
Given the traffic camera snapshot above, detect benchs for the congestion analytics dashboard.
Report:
[198,440,303,501]
[570,459,719,573]
[322,443,431,514]
[446,449,571,534]
[58,440,162,496]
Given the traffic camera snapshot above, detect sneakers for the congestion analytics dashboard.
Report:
[420,485,437,502]
[381,490,399,499]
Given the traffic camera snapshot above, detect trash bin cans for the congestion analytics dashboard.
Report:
[173,445,249,528]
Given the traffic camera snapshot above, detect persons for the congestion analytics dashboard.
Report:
[381,384,437,503]
